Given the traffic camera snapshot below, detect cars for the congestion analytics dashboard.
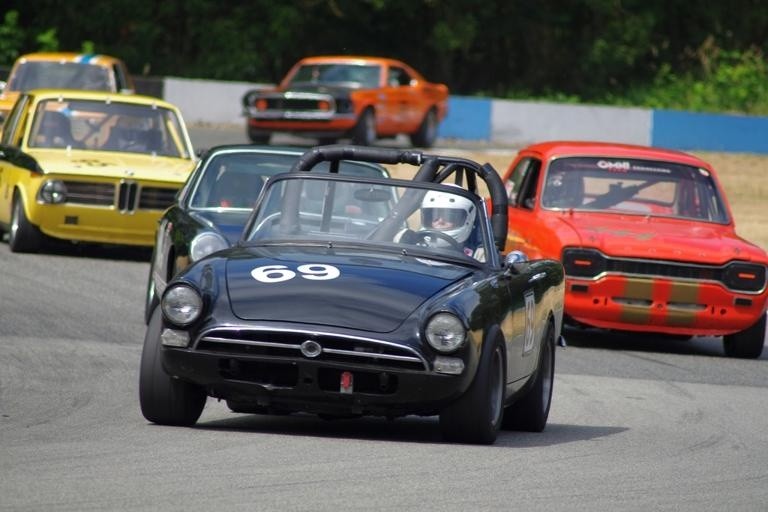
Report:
[481,140,767,358]
[0,53,134,149]
[240,56,449,148]
[144,143,410,326]
[0,88,211,252]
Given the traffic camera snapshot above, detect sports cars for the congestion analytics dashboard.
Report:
[140,144,567,444]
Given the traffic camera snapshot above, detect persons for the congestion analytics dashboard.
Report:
[394,183,487,265]
[116,115,156,156]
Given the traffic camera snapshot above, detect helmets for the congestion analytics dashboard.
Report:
[420,183,479,247]
